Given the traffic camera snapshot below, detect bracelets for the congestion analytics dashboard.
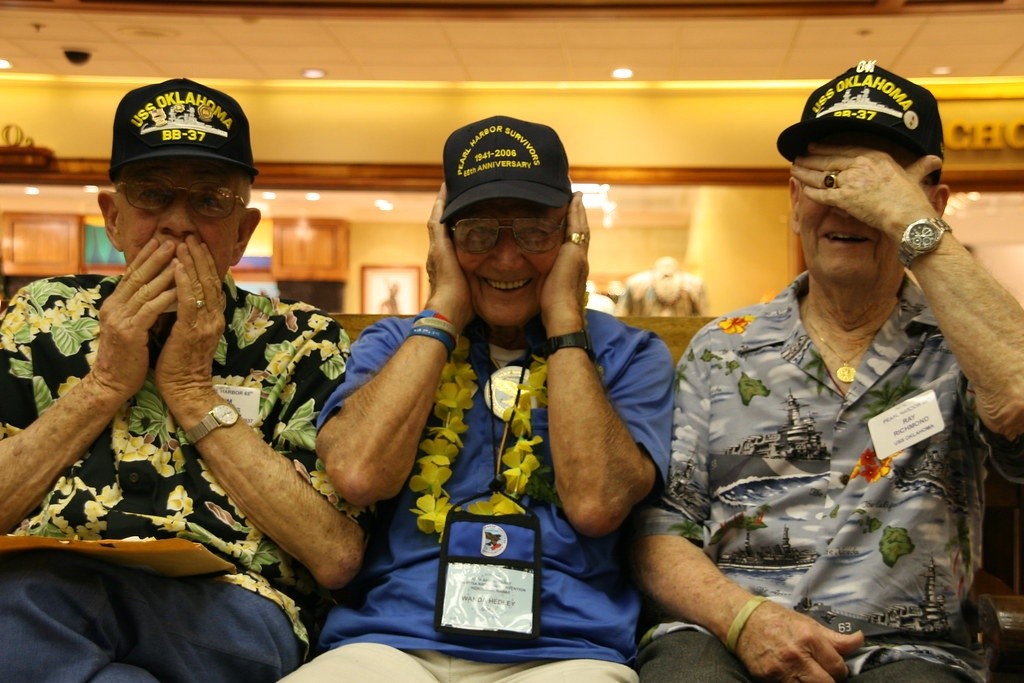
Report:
[726,595,768,653]
[408,309,457,363]
[545,330,597,362]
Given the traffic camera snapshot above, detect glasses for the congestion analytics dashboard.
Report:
[118,175,247,219]
[449,216,568,254]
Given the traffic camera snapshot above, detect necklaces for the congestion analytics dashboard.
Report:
[803,305,866,383]
[407,324,548,543]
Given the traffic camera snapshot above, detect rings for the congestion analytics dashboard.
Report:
[824,170,841,189]
[566,233,587,246]
[196,299,205,308]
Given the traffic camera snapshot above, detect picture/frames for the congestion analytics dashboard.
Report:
[361,265,424,316]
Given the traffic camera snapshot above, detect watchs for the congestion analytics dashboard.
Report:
[186,404,242,445]
[898,217,953,270]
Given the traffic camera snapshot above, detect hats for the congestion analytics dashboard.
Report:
[441,115,573,223]
[777,60,943,186]
[109,78,259,182]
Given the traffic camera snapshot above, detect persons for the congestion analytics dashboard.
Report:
[635,63,1024,683]
[278,117,675,683]
[1,78,370,683]
